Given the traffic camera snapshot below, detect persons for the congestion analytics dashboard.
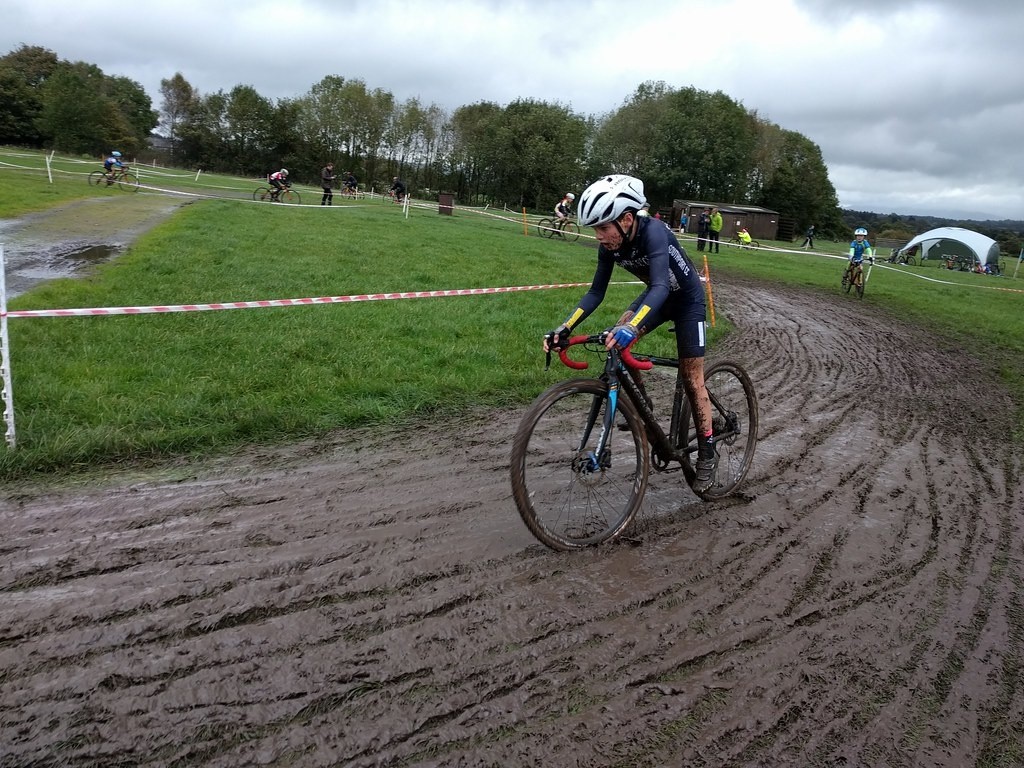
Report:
[388,177,404,201]
[551,193,575,236]
[320,162,338,206]
[104,151,128,186]
[655,207,723,253]
[344,172,358,198]
[269,168,289,202]
[736,227,752,249]
[801,225,815,249]
[841,228,873,289]
[542,174,721,492]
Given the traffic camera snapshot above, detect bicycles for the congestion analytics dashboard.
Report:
[251,182,301,206]
[842,256,875,300]
[537,212,580,242]
[341,184,365,201]
[383,189,410,207]
[728,235,760,252]
[939,254,1001,276]
[509,332,760,551]
[877,251,917,267]
[88,165,140,192]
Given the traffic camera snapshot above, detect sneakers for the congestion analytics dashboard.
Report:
[693,451,720,492]
[617,400,652,430]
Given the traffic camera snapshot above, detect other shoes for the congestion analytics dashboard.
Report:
[856,282,862,286]
[842,276,847,285]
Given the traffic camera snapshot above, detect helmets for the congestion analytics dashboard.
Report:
[577,174,647,228]
[566,193,575,200]
[855,228,868,237]
[280,169,288,175]
[112,151,121,157]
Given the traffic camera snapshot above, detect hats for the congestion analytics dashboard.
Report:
[704,207,710,212]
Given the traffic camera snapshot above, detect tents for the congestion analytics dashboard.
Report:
[893,227,1000,275]
